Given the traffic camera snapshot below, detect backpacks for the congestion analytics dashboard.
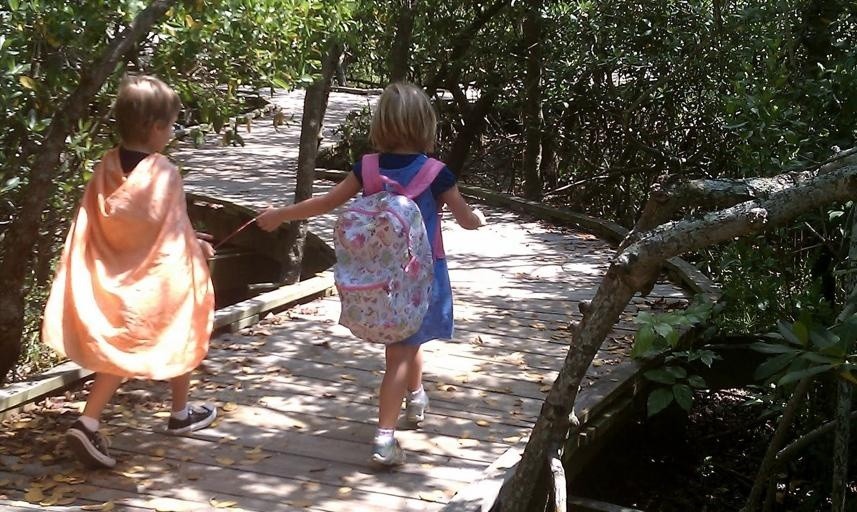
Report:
[332,153,446,345]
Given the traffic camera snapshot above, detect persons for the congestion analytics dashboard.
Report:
[34,74,221,470]
[257,81,486,467]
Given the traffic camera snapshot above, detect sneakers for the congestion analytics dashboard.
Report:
[167,402,217,433]
[371,436,407,466]
[64,420,117,468]
[406,392,430,422]
